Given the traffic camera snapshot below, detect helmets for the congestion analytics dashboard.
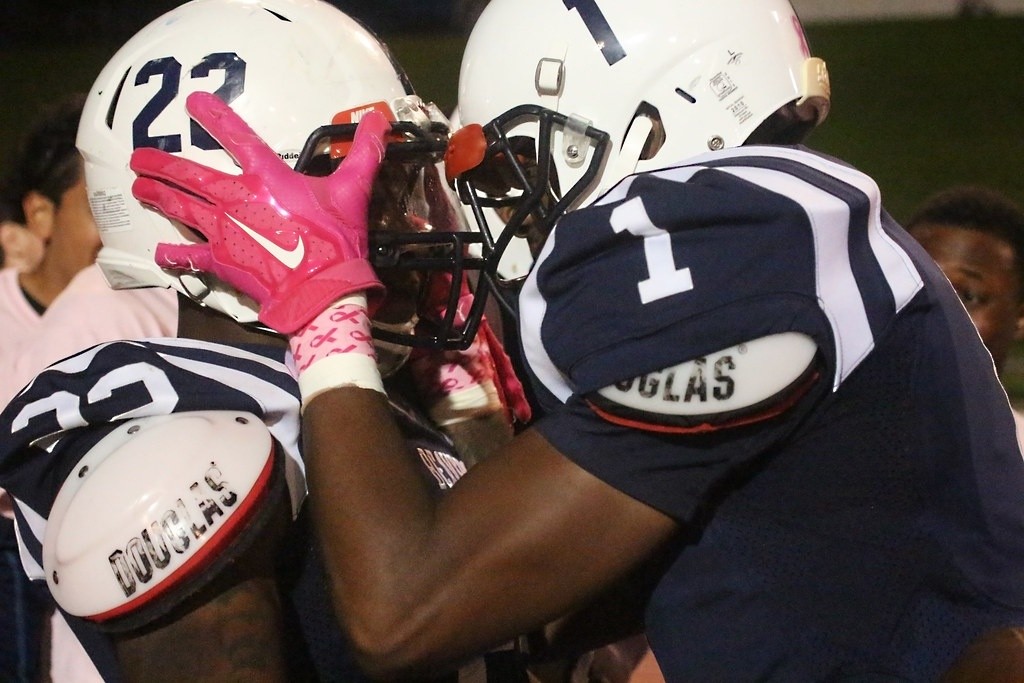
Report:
[72,1,446,328]
[446,0,832,210]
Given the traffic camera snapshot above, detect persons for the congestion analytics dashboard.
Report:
[0,1,452,683]
[132,2,1024,682]
[906,187,1024,460]
[0,96,178,409]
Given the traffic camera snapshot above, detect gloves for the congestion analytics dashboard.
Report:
[127,90,394,339]
